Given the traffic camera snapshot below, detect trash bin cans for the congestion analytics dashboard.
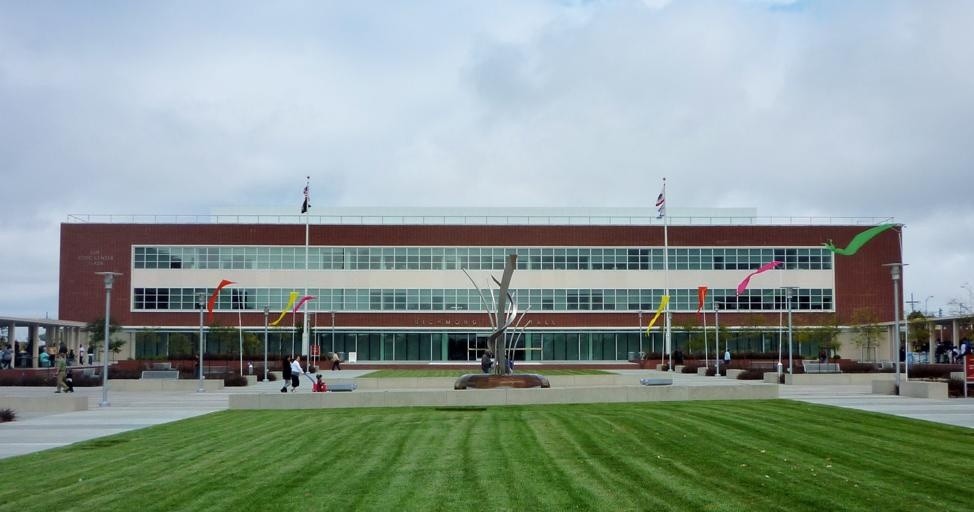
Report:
[337,352,344,362]
[627,352,636,361]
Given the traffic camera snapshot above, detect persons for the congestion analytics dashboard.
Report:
[290,355,304,392]
[193,354,200,378]
[281,355,291,392]
[724,349,730,364]
[481,350,494,373]
[934,337,972,364]
[316,373,325,390]
[332,351,341,371]
[0,340,95,393]
[505,355,513,373]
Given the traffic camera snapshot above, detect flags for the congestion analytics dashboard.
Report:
[655,190,665,218]
[301,187,311,213]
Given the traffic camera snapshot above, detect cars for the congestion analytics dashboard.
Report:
[18,341,67,369]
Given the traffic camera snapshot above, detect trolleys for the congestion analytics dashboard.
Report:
[304,373,357,392]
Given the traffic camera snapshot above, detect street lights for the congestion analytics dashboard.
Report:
[925,295,933,315]
[263,304,270,378]
[95,271,124,401]
[195,292,207,386]
[668,312,672,370]
[638,311,643,357]
[960,280,974,305]
[331,312,336,359]
[881,263,911,388]
[780,286,799,374]
[714,303,719,373]
[306,312,311,372]
